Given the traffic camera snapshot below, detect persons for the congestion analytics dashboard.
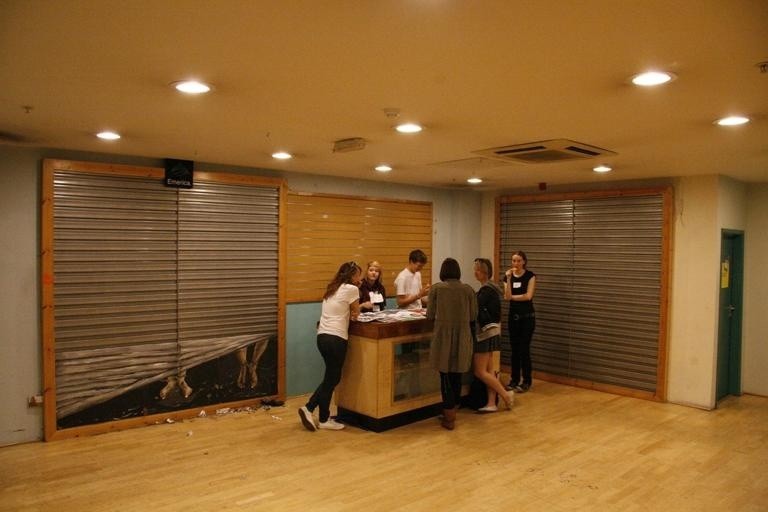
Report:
[503,251,536,393]
[427,258,478,430]
[237,338,269,388]
[359,261,386,312]
[298,261,362,431]
[394,250,432,309]
[474,258,514,412]
[160,370,192,400]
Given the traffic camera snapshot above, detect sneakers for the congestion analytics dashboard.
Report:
[505,380,530,393]
[478,406,498,412]
[298,406,317,432]
[506,390,514,409]
[317,417,345,430]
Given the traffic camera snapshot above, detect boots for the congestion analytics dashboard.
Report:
[441,408,456,430]
